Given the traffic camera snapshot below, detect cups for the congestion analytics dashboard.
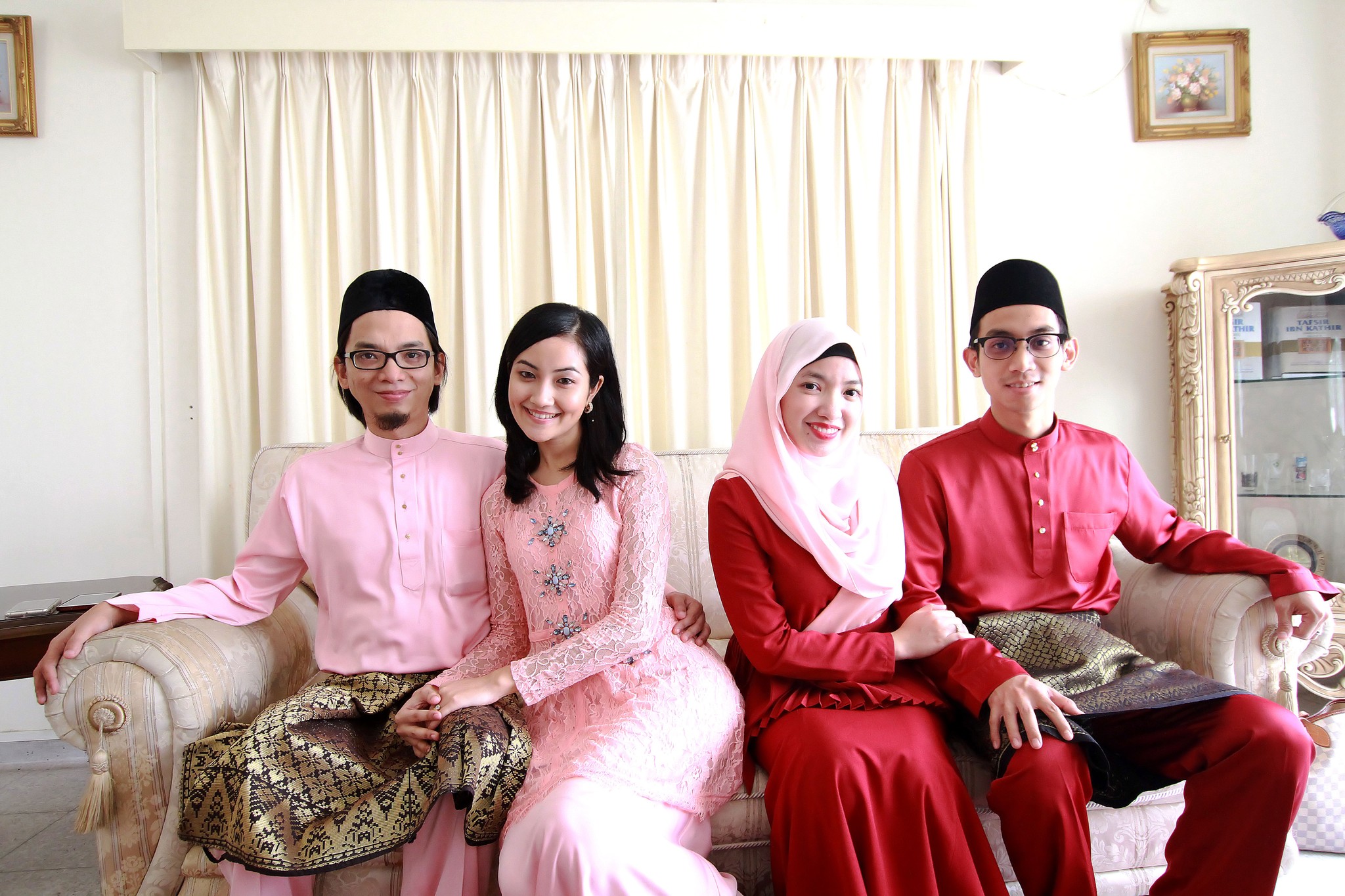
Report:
[1239,453,1259,490]
[1260,453,1287,480]
[1309,468,1331,493]
[1288,452,1308,485]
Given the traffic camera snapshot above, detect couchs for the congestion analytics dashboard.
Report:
[46,427,1335,896]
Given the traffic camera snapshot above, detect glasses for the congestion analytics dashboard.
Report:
[973,333,1068,360]
[341,348,437,370]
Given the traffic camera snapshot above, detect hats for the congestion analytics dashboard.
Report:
[337,269,438,346]
[970,259,1069,335]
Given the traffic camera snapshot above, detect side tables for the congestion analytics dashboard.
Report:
[0,575,174,681]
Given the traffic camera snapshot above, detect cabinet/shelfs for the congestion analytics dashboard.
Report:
[1160,239,1345,584]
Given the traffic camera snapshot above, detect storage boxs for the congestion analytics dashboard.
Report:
[1233,301,1262,380]
[1265,305,1345,379]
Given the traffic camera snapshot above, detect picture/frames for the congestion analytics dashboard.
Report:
[1131,29,1250,141]
[0,15,38,137]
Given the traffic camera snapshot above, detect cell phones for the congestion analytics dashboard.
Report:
[3,598,61,618]
[55,593,121,611]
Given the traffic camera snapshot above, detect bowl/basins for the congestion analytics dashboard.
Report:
[1317,211,1345,240]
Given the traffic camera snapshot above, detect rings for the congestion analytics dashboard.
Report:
[952,624,959,632]
[433,704,441,711]
[1315,630,1323,635]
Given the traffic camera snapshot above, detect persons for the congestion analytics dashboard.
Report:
[394,302,750,896]
[33,269,711,896]
[897,258,1340,896]
[701,317,1015,896]
